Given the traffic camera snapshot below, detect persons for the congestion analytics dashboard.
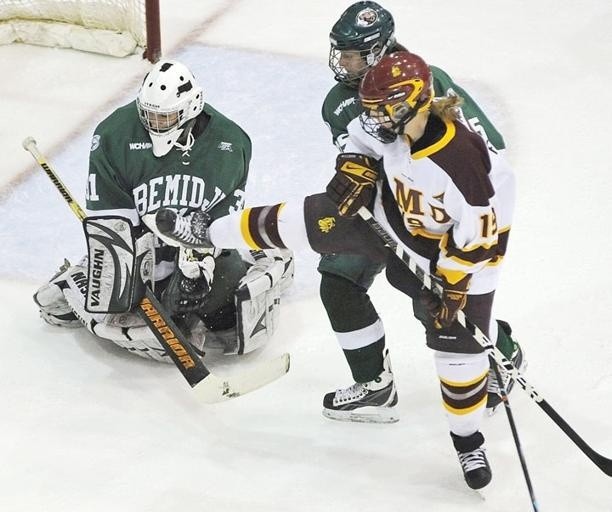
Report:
[33,60,252,364]
[156,51,499,490]
[321,0,523,412]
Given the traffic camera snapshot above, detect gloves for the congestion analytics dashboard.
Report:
[417,267,472,332]
[325,153,382,221]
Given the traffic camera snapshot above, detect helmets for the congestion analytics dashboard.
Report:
[329,0,395,68]
[135,61,206,136]
[358,51,436,113]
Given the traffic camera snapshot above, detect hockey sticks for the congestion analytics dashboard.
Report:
[22,135,288,403]
[354,205,612,476]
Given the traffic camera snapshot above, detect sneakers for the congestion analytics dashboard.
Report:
[487,319,523,408]
[155,207,214,249]
[322,349,398,411]
[448,433,492,491]
